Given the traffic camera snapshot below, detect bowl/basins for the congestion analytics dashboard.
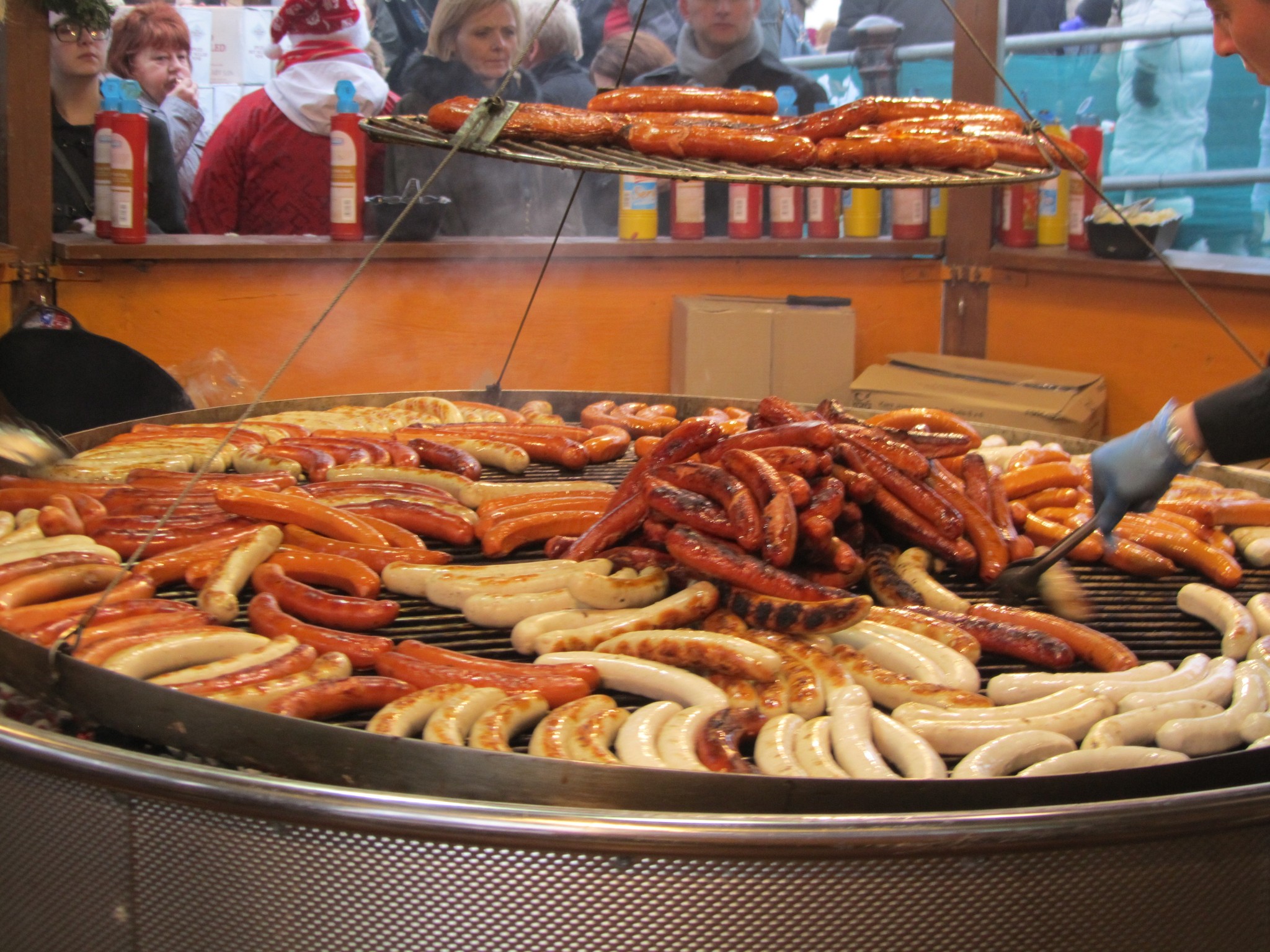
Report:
[367,179,453,240]
[1084,204,1185,258]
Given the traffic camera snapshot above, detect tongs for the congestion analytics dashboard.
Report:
[997,511,1102,609]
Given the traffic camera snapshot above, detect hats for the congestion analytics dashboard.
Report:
[264,0,371,60]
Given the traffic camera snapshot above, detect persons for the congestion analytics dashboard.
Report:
[1088,3,1270,552]
[45,0,1270,263]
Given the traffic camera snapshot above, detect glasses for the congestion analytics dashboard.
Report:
[49,18,111,43]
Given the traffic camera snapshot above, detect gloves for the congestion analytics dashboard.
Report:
[1132,67,1160,109]
[1090,396,1199,554]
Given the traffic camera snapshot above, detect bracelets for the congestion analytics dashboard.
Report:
[1157,401,1203,467]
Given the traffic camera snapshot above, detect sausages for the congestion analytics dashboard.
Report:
[427,82,1088,172]
[0,389,1268,776]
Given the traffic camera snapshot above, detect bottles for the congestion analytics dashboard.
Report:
[608,122,1112,246]
[87,69,152,243]
[323,80,367,240]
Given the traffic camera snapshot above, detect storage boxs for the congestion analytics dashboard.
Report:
[672,295,855,407]
[847,348,1108,441]
[1087,212,1184,262]
[364,179,452,242]
